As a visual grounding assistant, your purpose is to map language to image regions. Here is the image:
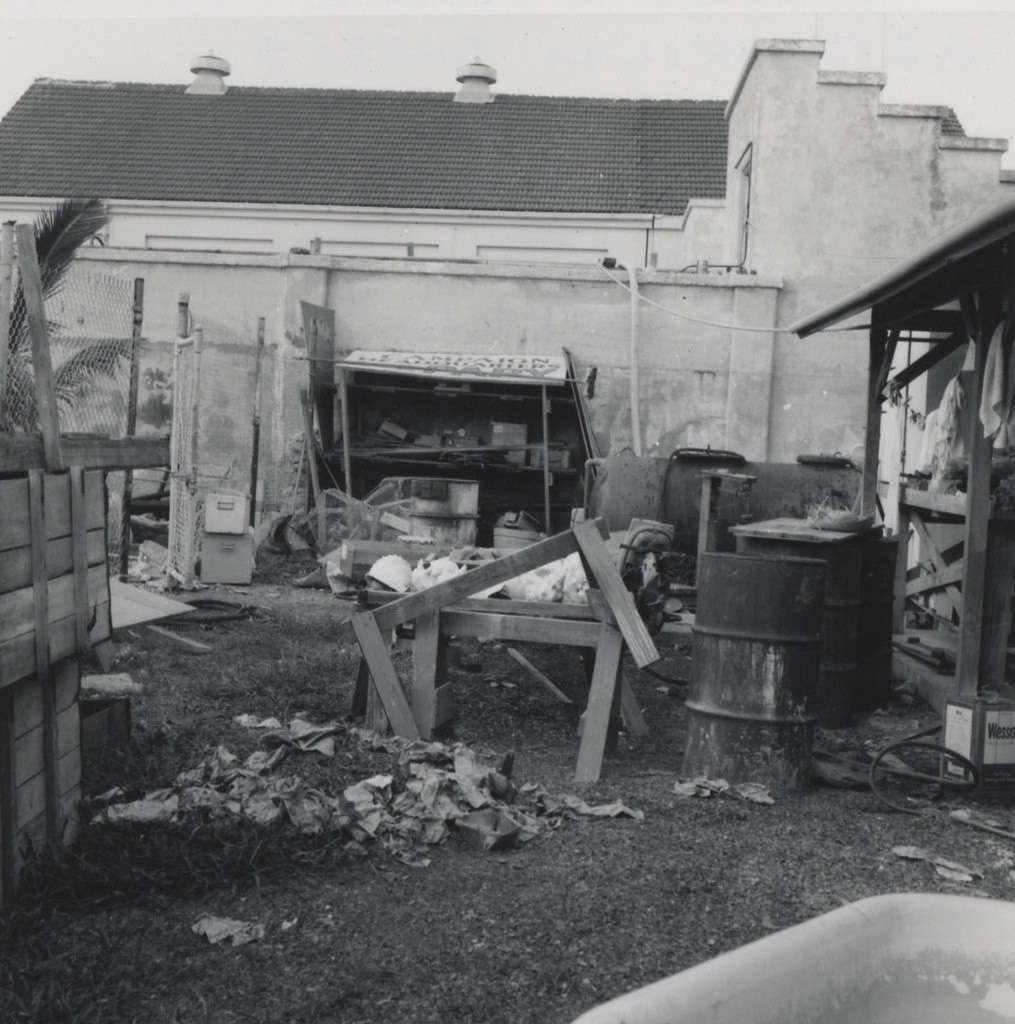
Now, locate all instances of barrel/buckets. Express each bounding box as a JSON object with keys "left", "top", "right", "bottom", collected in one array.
[
  {"left": 857, "top": 531, "right": 901, "bottom": 710},
  {"left": 679, "top": 551, "right": 823, "bottom": 800},
  {"left": 734, "top": 531, "right": 867, "bottom": 730}
]
